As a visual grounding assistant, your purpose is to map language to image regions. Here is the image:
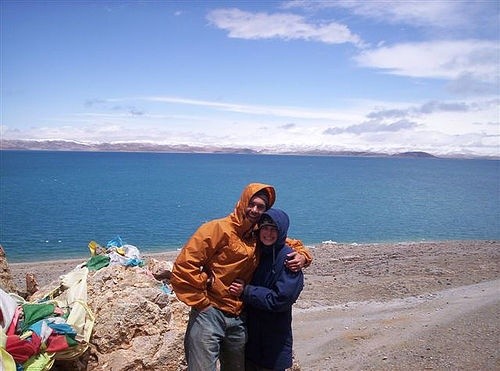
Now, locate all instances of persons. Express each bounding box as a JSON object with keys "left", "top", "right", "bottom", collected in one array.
[
  {"left": 229, "top": 209, "right": 304, "bottom": 371},
  {"left": 171, "top": 183, "right": 313, "bottom": 371}
]
